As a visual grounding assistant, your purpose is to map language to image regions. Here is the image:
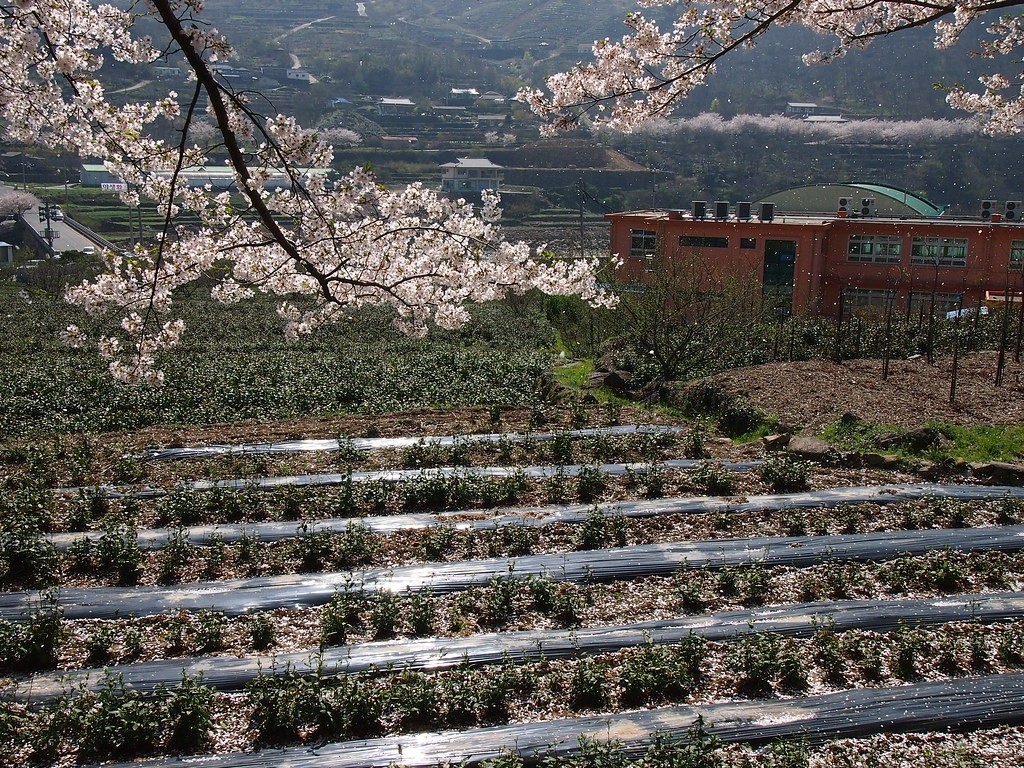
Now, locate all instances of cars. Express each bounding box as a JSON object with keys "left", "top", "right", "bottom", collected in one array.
[
  {"left": 51, "top": 209, "right": 64, "bottom": 221},
  {"left": 49, "top": 205, "right": 62, "bottom": 212},
  {"left": 83, "top": 247, "right": 97, "bottom": 255}
]
